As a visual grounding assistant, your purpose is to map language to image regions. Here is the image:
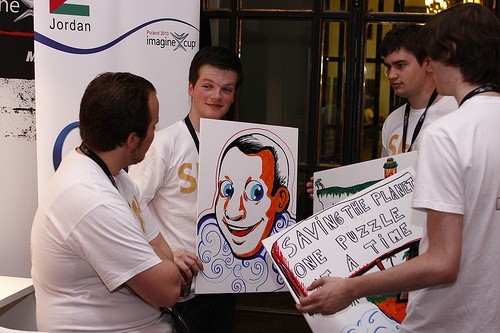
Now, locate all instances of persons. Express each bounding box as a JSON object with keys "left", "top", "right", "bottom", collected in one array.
[
  {"left": 128, "top": 45, "right": 242, "bottom": 333},
  {"left": 30, "top": 72, "right": 181, "bottom": 333},
  {"left": 294, "top": 3, "right": 500, "bottom": 333},
  {"left": 307, "top": 23, "right": 459, "bottom": 198}
]
